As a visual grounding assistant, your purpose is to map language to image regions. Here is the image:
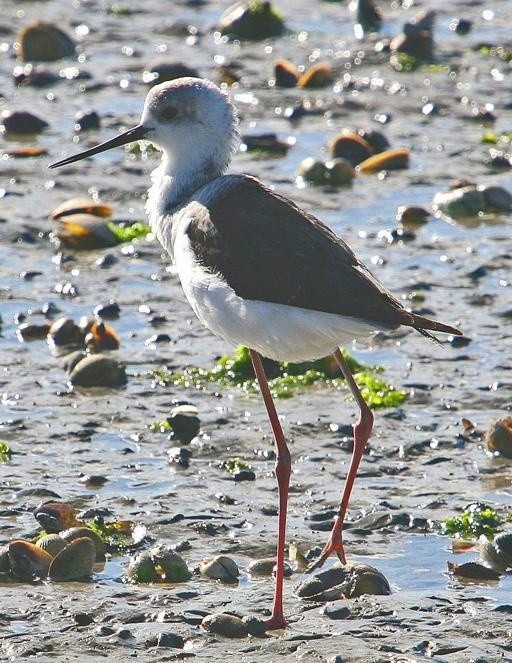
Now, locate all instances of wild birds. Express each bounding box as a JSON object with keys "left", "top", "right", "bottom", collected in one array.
[{"left": 45, "top": 75, "right": 463, "bottom": 635}]
